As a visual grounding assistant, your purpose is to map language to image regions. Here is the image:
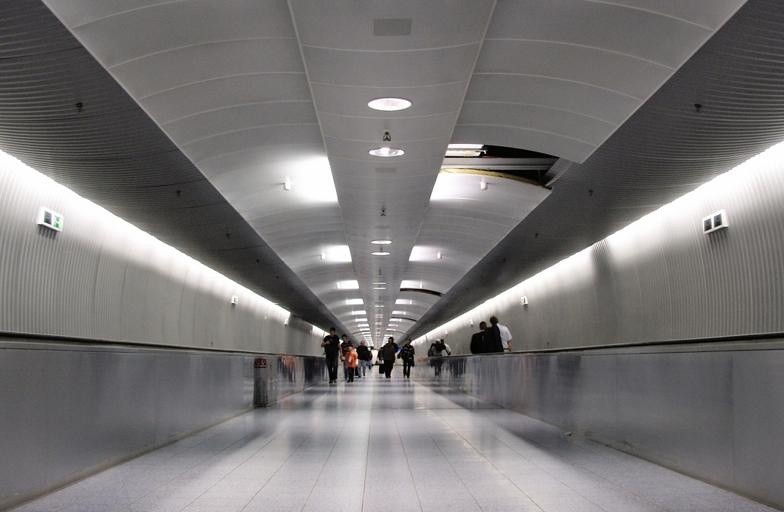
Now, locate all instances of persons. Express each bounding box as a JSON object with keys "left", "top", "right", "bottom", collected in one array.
[
  {"left": 487, "top": 317, "right": 513, "bottom": 352},
  {"left": 470, "top": 322, "right": 487, "bottom": 354},
  {"left": 321, "top": 327, "right": 451, "bottom": 384}
]
